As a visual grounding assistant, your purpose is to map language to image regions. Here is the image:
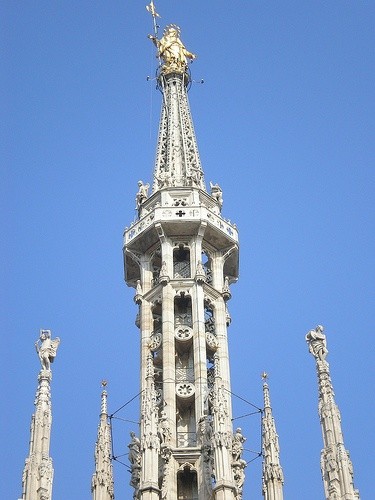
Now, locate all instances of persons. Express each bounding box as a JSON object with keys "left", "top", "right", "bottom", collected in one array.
[
  {"left": 35, "top": 333, "right": 54, "bottom": 370},
  {"left": 146, "top": 27, "right": 195, "bottom": 67},
  {"left": 308, "top": 325, "right": 329, "bottom": 362},
  {"left": 232, "top": 427, "right": 247, "bottom": 461},
  {"left": 154, "top": 407, "right": 218, "bottom": 458},
  {"left": 128, "top": 431, "right": 141, "bottom": 465}
]
